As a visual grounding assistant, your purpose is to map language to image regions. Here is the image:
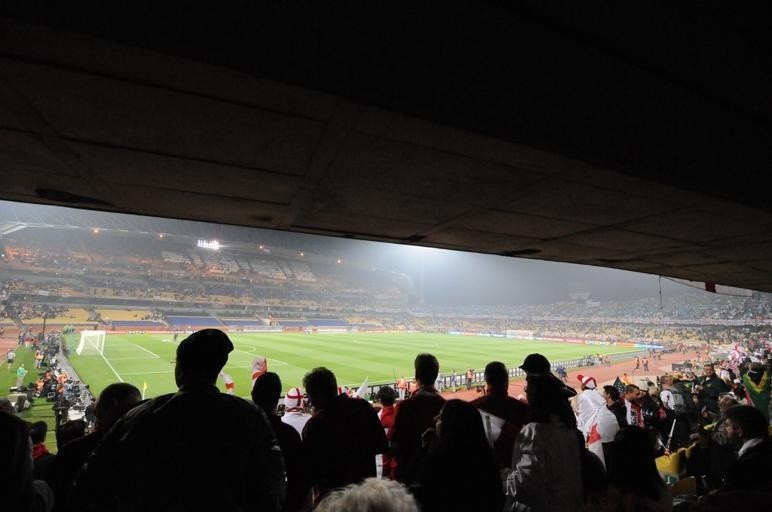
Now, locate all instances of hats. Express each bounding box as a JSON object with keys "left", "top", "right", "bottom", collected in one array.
[
  {"left": 576, "top": 374, "right": 598, "bottom": 391},
  {"left": 517, "top": 352, "right": 552, "bottom": 374},
  {"left": 176, "top": 327, "right": 232, "bottom": 376},
  {"left": 283, "top": 386, "right": 305, "bottom": 409}
]
